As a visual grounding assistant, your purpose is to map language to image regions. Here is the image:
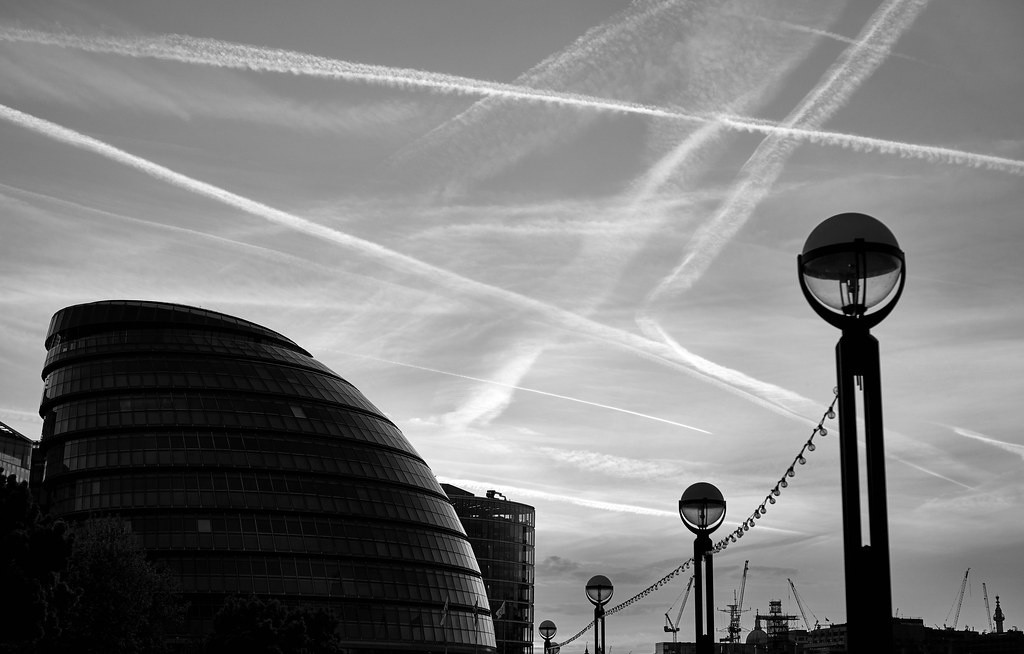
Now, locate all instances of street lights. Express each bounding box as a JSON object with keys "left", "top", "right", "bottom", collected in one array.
[
  {"left": 538, "top": 620, "right": 557, "bottom": 654},
  {"left": 584, "top": 575, "right": 614, "bottom": 653},
  {"left": 792, "top": 213, "right": 908, "bottom": 654},
  {"left": 678, "top": 481, "right": 728, "bottom": 654}
]
